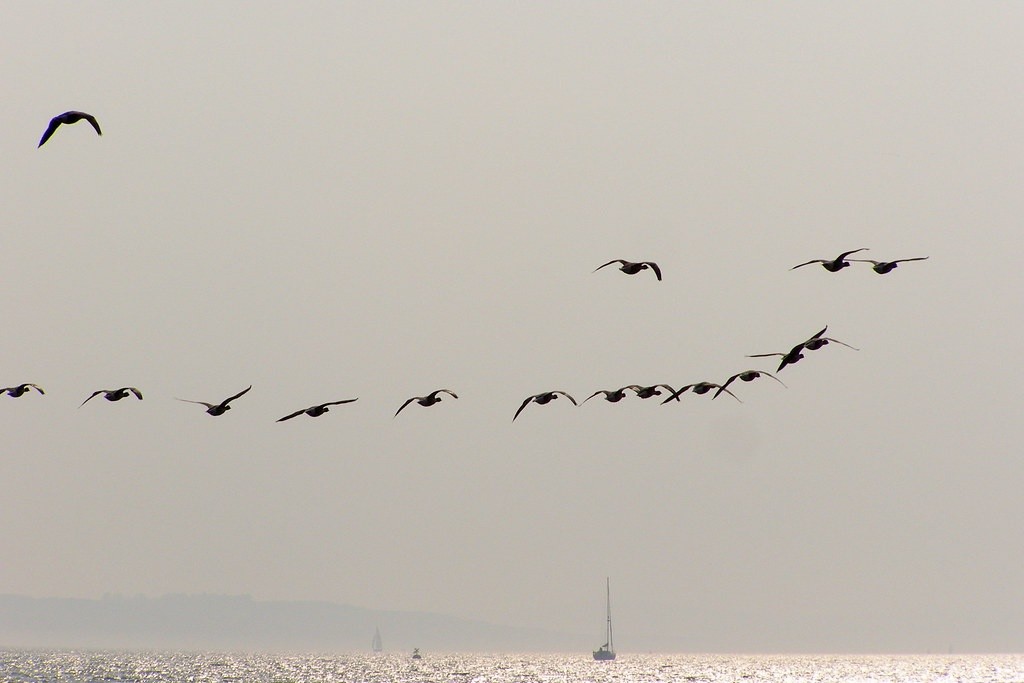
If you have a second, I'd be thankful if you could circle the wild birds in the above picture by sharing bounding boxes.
[591,259,662,281]
[711,370,788,400]
[628,383,681,403]
[37,111,101,149]
[659,381,743,405]
[512,390,578,423]
[0,383,45,398]
[843,255,930,275]
[775,338,859,374]
[78,386,143,409]
[744,325,828,373]
[579,384,642,406]
[174,383,252,416]
[788,248,870,272]
[395,388,459,416]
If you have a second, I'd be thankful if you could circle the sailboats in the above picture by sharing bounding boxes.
[372,626,383,652]
[592,576,617,660]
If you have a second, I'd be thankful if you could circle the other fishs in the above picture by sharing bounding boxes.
[276,397,360,423]
